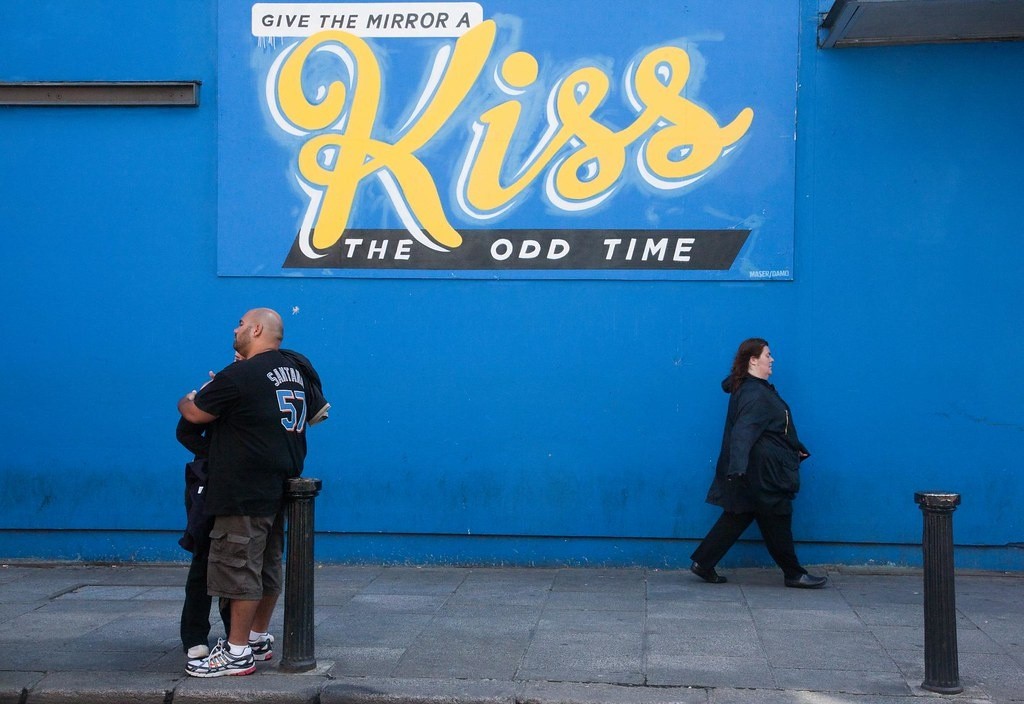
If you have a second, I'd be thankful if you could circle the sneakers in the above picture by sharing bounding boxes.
[184,637,256,677]
[187,644,210,658]
[269,634,275,643]
[217,636,272,660]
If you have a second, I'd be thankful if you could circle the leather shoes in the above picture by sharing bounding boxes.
[785,572,827,589]
[690,561,726,583]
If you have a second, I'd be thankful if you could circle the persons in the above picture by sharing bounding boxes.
[690,338,827,589]
[176,308,307,677]
